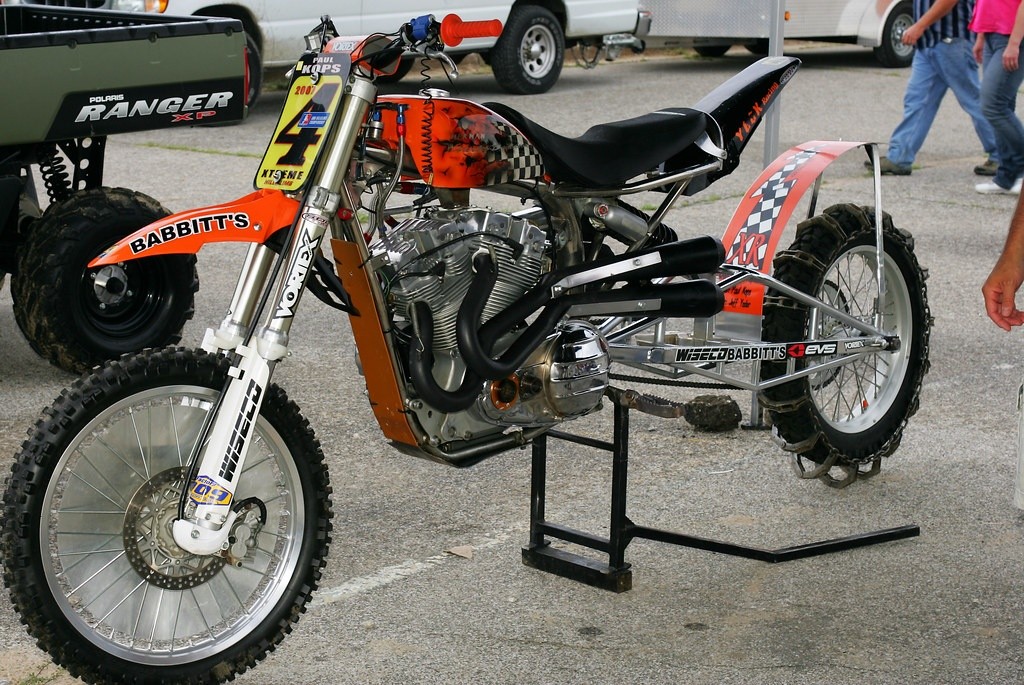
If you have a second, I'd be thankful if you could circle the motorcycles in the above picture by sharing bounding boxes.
[1,15,940,685]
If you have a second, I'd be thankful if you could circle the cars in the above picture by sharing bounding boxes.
[114,1,657,128]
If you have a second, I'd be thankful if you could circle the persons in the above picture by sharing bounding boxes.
[863,0,999,176]
[980,178,1024,332]
[969,0,1024,194]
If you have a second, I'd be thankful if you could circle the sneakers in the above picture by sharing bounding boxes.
[864,156,912,175]
[974,159,1000,175]
[975,177,1023,195]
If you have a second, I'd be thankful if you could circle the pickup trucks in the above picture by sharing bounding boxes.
[1,0,253,378]
